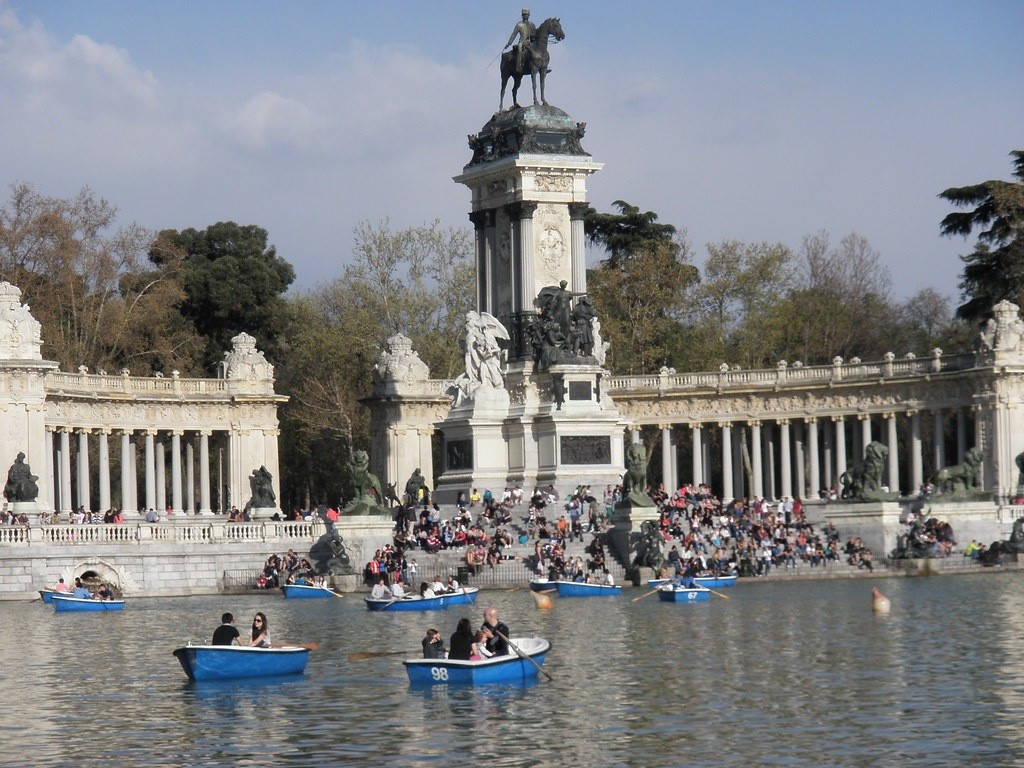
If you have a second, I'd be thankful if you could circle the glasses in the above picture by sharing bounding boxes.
[254,618,261,622]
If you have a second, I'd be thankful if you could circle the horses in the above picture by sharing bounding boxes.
[499,17,565,115]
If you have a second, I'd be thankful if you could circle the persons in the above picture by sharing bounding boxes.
[247,612,272,649]
[901,511,953,556]
[0,506,123,540]
[919,480,934,500]
[453,308,504,407]
[422,608,509,663]
[247,465,276,507]
[880,483,889,494]
[965,540,987,557]
[506,8,551,73]
[141,505,173,534]
[1007,494,1024,506]
[212,612,243,646]
[534,279,598,367]
[406,468,427,504]
[362,483,874,601]
[840,467,862,498]
[292,505,340,523]
[385,482,402,508]
[1010,516,1024,543]
[543,228,560,249]
[5,452,39,501]
[55,576,114,602]
[226,504,251,536]
[257,547,328,591]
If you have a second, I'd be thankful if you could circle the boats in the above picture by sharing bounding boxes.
[172,646,311,680]
[280,585,334,598]
[528,579,557,592]
[50,596,125,611]
[402,635,552,685]
[554,581,623,597]
[656,587,711,603]
[402,587,480,605]
[38,589,94,604]
[647,575,737,589]
[364,594,449,611]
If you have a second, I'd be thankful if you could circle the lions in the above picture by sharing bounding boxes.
[340,449,384,516]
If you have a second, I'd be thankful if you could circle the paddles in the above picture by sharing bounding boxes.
[632,582,675,601]
[459,581,474,604]
[495,629,553,681]
[316,582,344,597]
[506,578,533,592]
[368,649,423,658]
[377,591,412,612]
[538,588,557,594]
[93,585,109,611]
[28,597,41,603]
[263,642,319,651]
[597,584,622,586]
[701,585,728,599]
